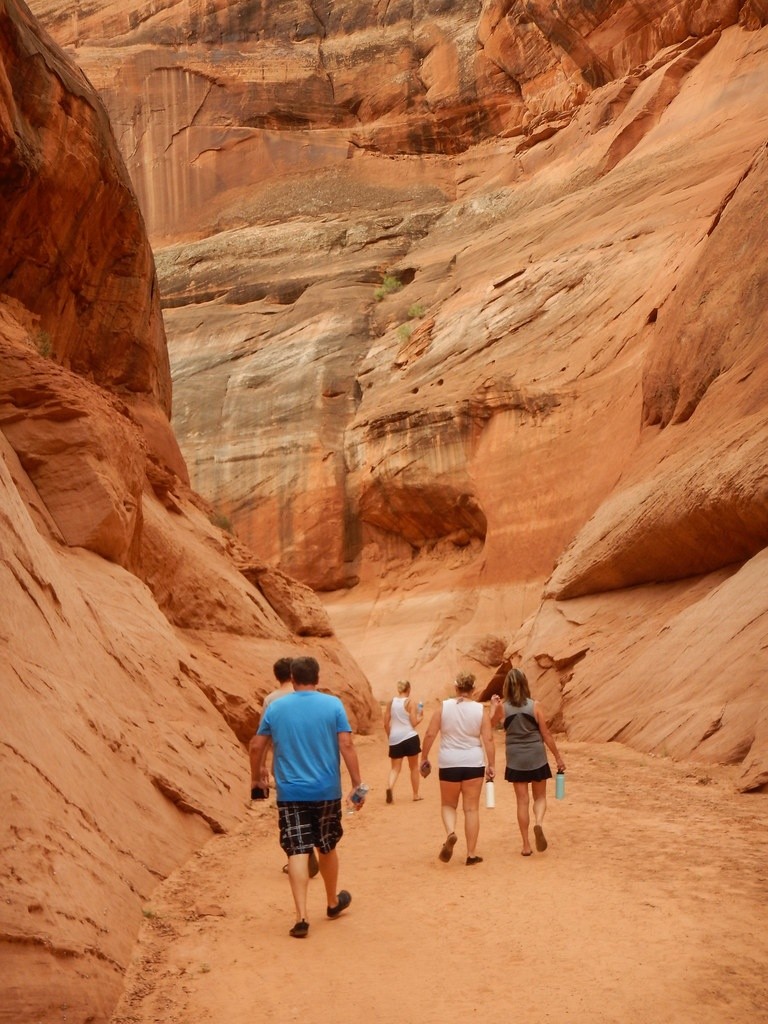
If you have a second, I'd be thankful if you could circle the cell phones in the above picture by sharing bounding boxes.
[422,763,431,778]
[251,787,269,799]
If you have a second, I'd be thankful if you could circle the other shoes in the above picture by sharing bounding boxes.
[327,890,351,917]
[413,796,424,800]
[289,918,309,936]
[386,789,392,803]
[440,832,458,862]
[466,856,483,865]
[308,852,319,878]
[283,864,288,873]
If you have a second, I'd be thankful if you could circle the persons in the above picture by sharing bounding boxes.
[419,671,496,866]
[490,668,567,856]
[384,680,424,804]
[249,656,365,937]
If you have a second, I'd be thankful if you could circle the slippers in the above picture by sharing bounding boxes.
[521,849,534,856]
[534,825,547,853]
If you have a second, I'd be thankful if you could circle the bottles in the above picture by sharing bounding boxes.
[556,769,564,800]
[346,784,368,814]
[486,778,495,808]
[417,701,423,718]
